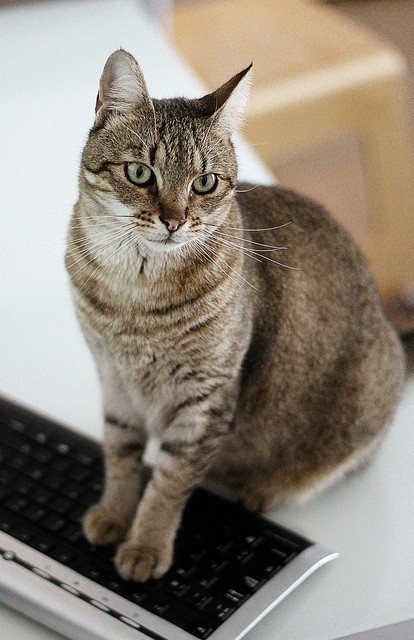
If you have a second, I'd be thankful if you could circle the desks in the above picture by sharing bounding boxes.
[0,0,413,638]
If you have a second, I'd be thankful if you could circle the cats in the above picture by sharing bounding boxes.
[62,45,411,589]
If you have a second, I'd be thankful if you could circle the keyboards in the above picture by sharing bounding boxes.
[0,389,341,640]
